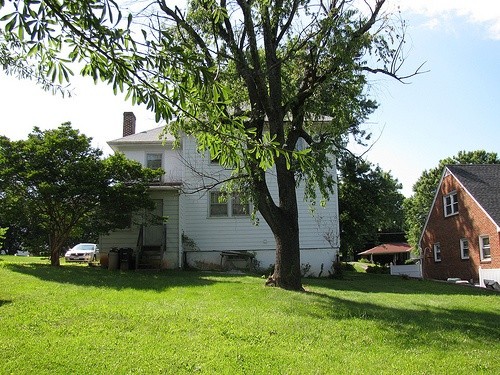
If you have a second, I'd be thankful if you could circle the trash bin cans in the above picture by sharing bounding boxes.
[107,247,119,271]
[118,247,134,272]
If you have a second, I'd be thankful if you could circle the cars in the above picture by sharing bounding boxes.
[65,243,100,263]
